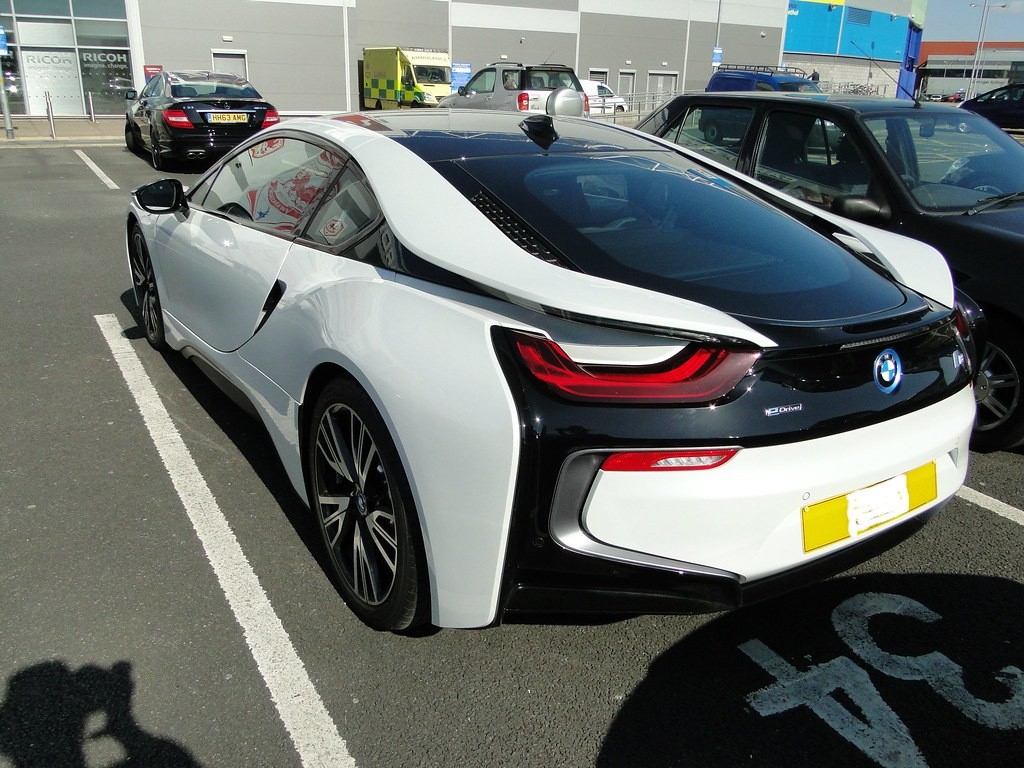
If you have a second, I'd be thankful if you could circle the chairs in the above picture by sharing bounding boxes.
[548,78,559,87]
[530,77,544,88]
[172,87,197,98]
[829,132,874,198]
[215,81,234,94]
[760,124,819,182]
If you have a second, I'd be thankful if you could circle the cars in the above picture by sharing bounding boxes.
[125,70,281,172]
[922,92,965,102]
[125,109,978,638]
[959,83,1024,128]
[102,78,134,100]
[4,72,23,100]
[633,91,1024,453]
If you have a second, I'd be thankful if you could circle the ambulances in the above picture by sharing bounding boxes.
[362,47,451,110]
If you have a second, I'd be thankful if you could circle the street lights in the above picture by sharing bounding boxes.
[963,1,1008,102]
[941,59,970,97]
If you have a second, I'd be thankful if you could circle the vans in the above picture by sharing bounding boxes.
[578,79,627,116]
[698,64,842,154]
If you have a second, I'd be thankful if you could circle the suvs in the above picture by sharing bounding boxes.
[436,62,590,122]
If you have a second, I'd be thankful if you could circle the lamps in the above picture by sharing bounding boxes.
[828,2,837,9]
[890,13,898,19]
